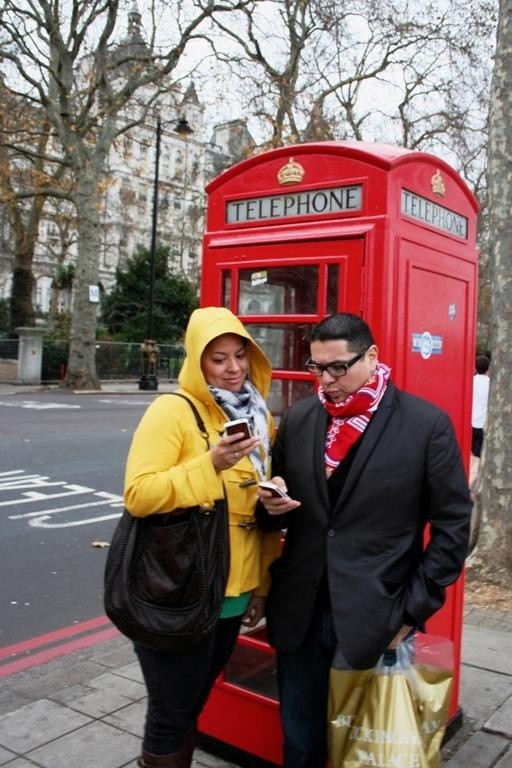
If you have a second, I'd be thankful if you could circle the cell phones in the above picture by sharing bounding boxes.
[224,419,252,447]
[259,482,293,502]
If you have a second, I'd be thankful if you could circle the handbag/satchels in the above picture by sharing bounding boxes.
[102,498,231,645]
[323,634,455,768]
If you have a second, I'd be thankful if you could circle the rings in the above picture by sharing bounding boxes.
[233,452,242,459]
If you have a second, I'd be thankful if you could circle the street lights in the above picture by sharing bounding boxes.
[147,114,194,340]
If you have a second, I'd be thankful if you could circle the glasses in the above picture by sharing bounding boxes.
[303,354,364,379]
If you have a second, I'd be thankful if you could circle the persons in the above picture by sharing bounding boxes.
[124,303,283,768]
[469,352,490,490]
[255,311,475,768]
